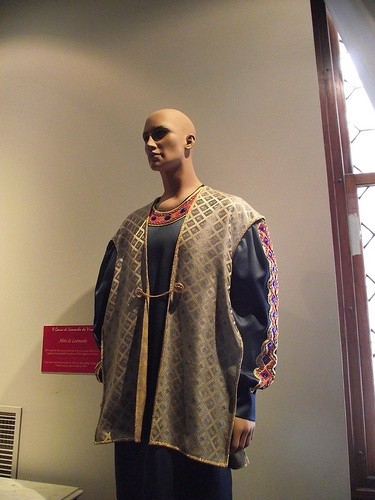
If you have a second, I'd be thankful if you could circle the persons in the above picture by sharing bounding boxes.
[90,109,280,500]
